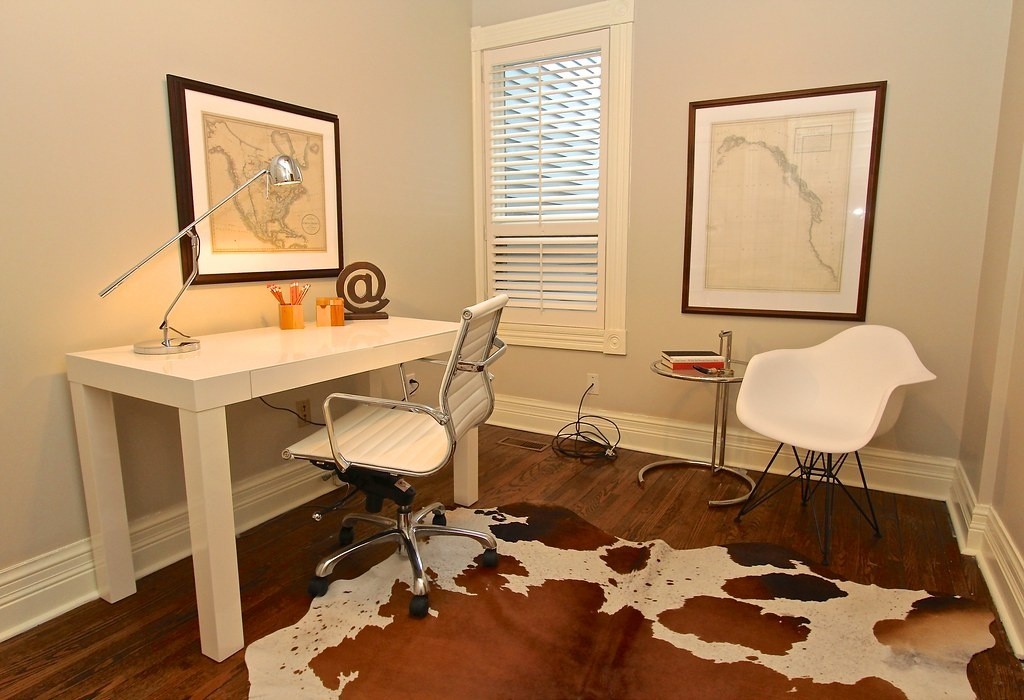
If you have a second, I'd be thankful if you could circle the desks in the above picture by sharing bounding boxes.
[637,328,757,507]
[66,315,480,663]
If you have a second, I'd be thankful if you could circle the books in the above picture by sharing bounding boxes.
[660,350,726,370]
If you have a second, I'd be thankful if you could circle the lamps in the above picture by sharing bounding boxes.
[100,153,303,356]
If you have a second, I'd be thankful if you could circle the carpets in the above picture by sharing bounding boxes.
[240,502,998,700]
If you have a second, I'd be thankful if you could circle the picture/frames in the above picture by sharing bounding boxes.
[680,78,889,324]
[165,72,344,285]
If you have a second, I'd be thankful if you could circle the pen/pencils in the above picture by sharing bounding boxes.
[289,281,312,306]
[267,282,285,306]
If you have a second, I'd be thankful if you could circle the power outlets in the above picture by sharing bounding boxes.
[405,373,417,395]
[587,373,599,395]
[295,398,312,427]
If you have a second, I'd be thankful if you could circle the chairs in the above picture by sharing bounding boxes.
[735,323,939,569]
[277,293,511,617]
